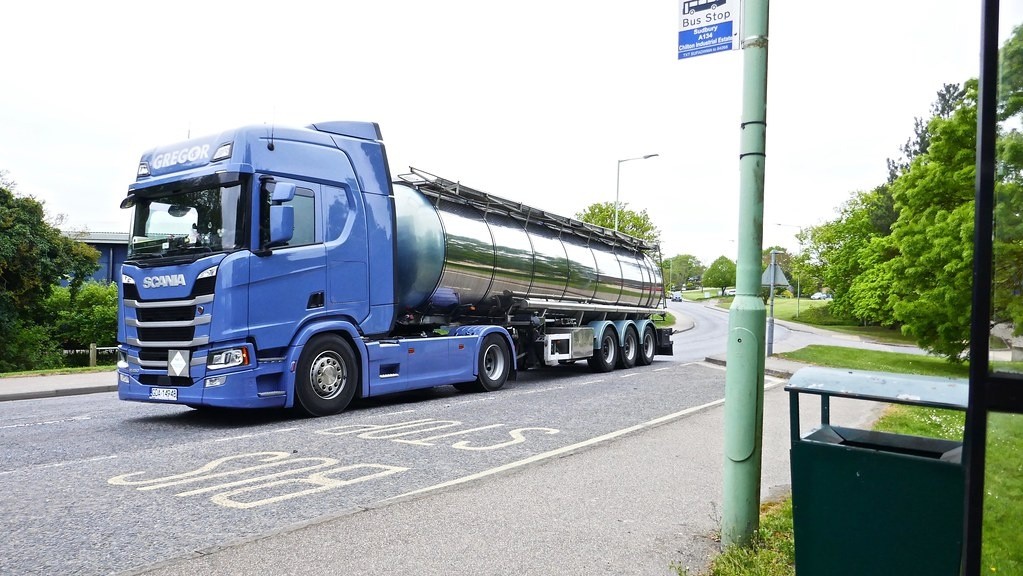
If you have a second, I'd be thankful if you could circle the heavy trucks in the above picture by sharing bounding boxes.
[115,119,675,417]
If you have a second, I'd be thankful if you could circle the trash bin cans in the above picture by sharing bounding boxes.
[783,365,970,576]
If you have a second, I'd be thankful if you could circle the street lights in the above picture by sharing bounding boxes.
[777,223,800,319]
[767,249,784,356]
[613,153,660,230]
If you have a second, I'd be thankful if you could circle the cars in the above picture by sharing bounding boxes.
[671,292,682,302]
[666,291,672,299]
[726,289,735,296]
[811,293,827,300]
